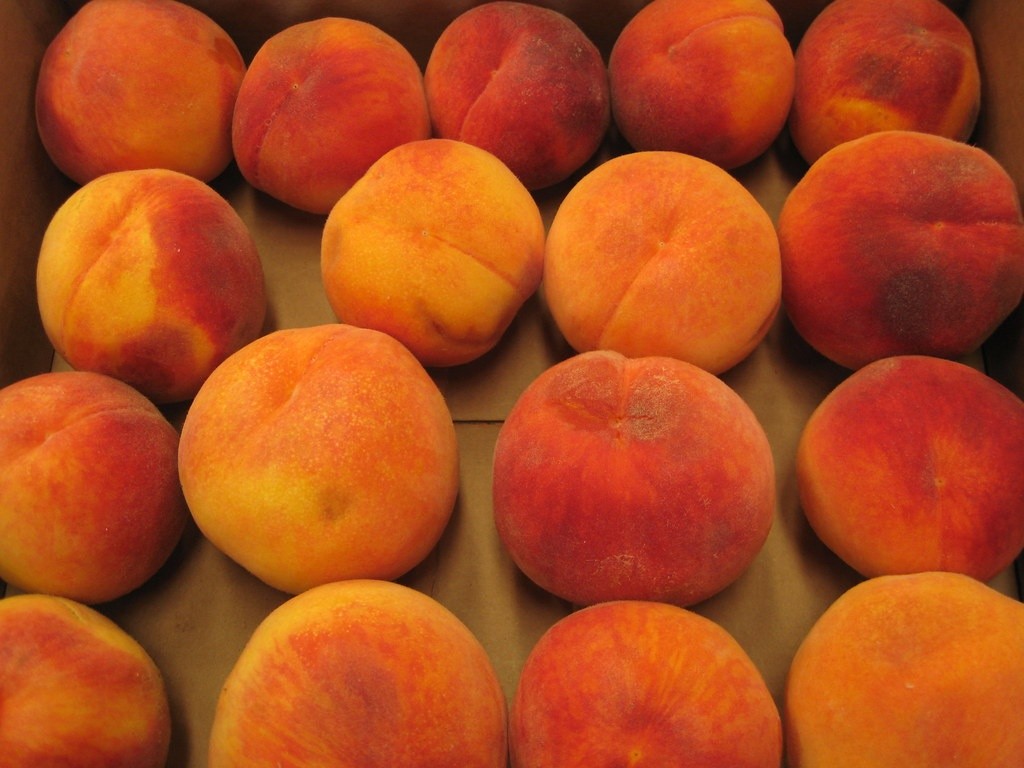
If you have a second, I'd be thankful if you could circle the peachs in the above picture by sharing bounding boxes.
[0,0,1024,768]
